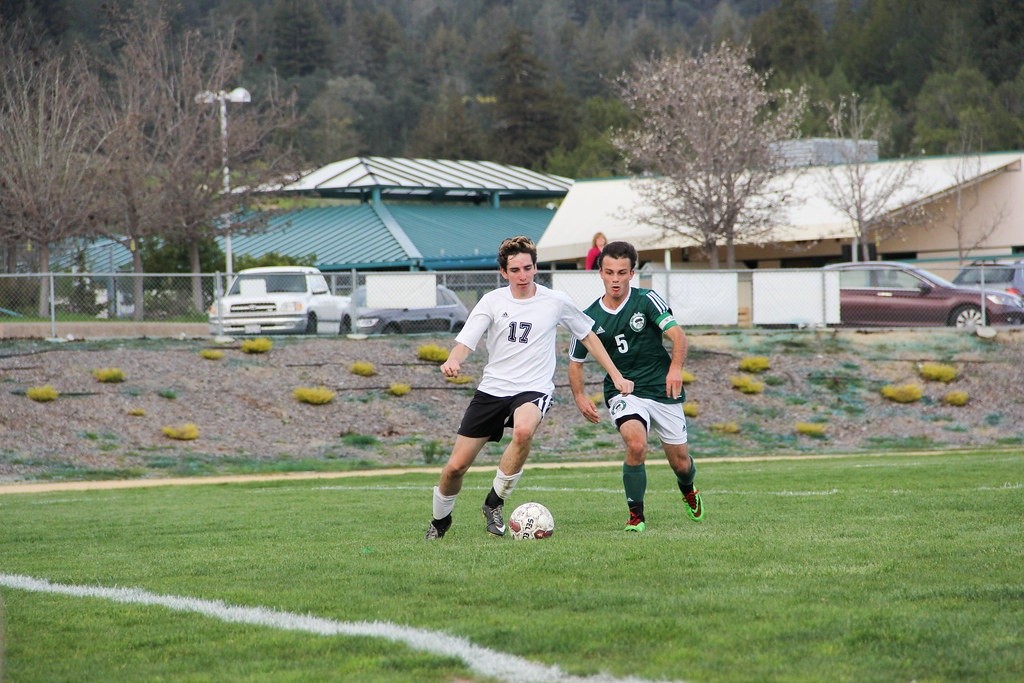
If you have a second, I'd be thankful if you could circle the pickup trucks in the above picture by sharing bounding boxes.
[209,263,351,338]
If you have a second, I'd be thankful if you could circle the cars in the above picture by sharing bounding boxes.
[338,280,470,336]
[952,257,1023,303]
[791,260,1024,331]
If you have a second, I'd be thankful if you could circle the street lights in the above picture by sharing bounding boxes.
[195,85,252,287]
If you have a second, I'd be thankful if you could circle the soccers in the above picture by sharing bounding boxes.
[508,502,555,541]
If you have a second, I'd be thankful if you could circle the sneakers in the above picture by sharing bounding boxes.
[682,485,704,522]
[482,502,506,536]
[624,514,645,533]
[424,516,453,541]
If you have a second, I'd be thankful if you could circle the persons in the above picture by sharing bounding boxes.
[424,234,635,542]
[585,232,607,270]
[568,241,703,534]
